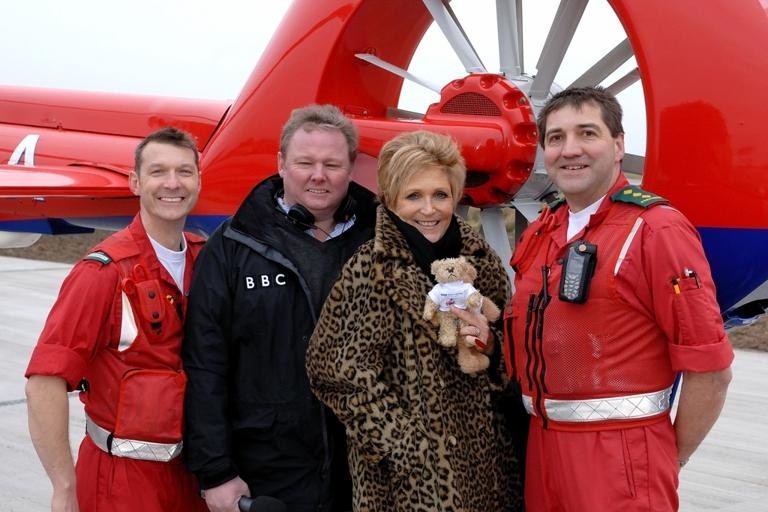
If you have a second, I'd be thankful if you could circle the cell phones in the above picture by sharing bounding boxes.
[563,251,586,301]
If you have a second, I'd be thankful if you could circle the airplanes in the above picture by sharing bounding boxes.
[0,0,768,335]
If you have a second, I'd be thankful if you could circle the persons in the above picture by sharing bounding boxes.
[177,96,389,512]
[23,129,210,512]
[490,86,737,510]
[302,130,522,512]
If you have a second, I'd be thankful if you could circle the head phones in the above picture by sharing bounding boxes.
[274,188,359,231]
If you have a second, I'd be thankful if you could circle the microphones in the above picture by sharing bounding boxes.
[198,489,287,512]
